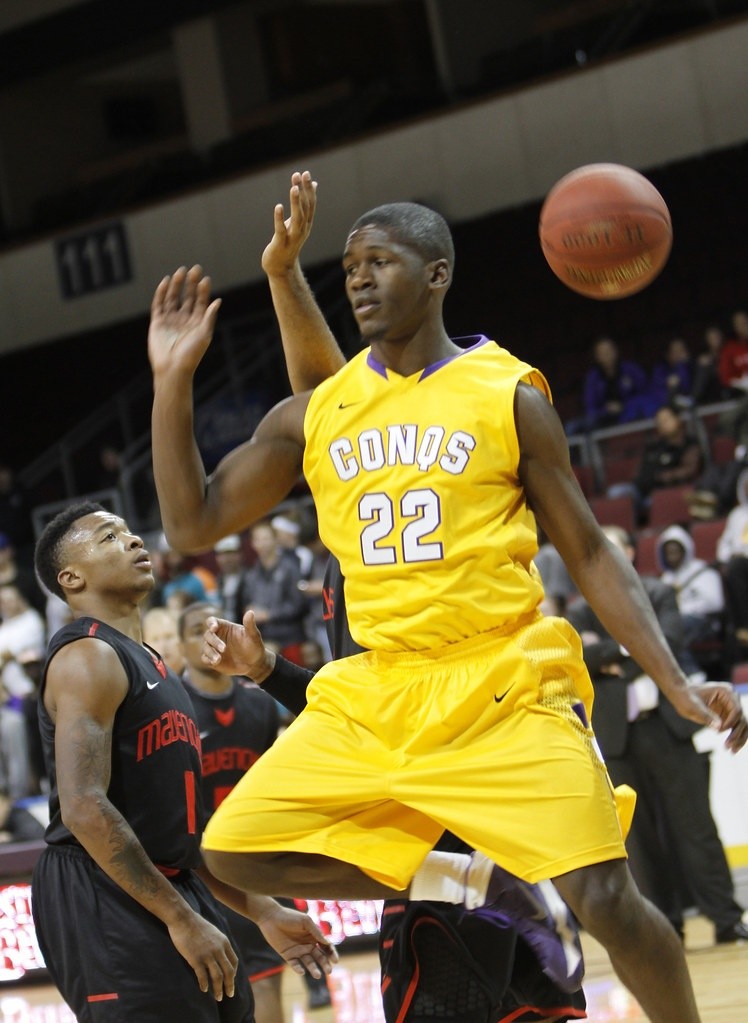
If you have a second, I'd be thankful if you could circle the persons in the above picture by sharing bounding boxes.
[201,170,588,1022]
[147,200,747,1023]
[0,311,747,1023]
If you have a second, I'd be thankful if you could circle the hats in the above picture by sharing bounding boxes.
[213,533,241,553]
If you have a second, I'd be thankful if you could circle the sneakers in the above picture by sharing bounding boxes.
[462,847,585,993]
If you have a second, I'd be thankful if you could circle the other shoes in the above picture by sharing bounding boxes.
[302,965,329,1009]
[716,919,748,944]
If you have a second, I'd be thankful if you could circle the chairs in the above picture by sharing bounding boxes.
[580,425,748,680]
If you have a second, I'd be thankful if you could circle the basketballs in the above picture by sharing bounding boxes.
[540,162,674,301]
